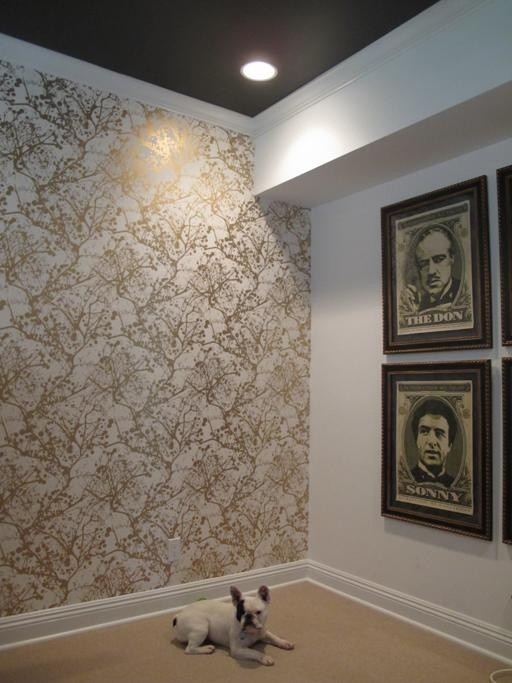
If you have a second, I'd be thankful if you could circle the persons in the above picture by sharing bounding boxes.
[398,226,462,313]
[403,401,465,489]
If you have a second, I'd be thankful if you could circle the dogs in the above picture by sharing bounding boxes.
[169,584,295,667]
